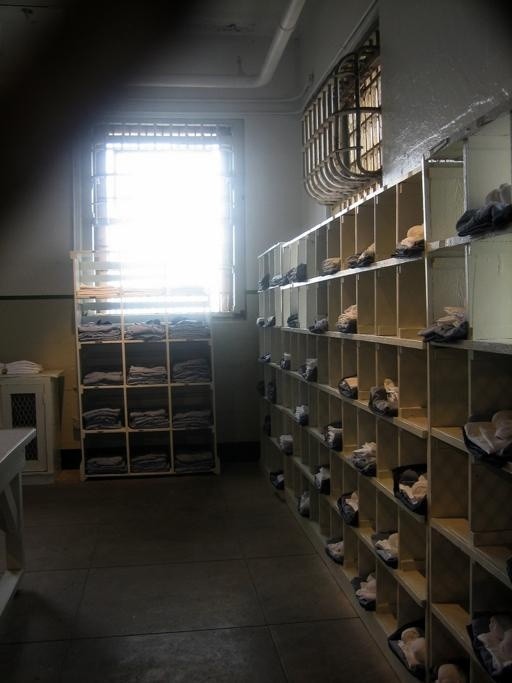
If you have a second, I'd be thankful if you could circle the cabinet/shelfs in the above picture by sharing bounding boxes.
[256,143,512,682]
[0,376,59,485]
[72,250,222,481]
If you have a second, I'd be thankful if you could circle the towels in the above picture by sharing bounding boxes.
[1,360,44,375]
[257,181,512,681]
[76,285,213,476]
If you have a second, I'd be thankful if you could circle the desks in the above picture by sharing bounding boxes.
[0,428,38,619]
